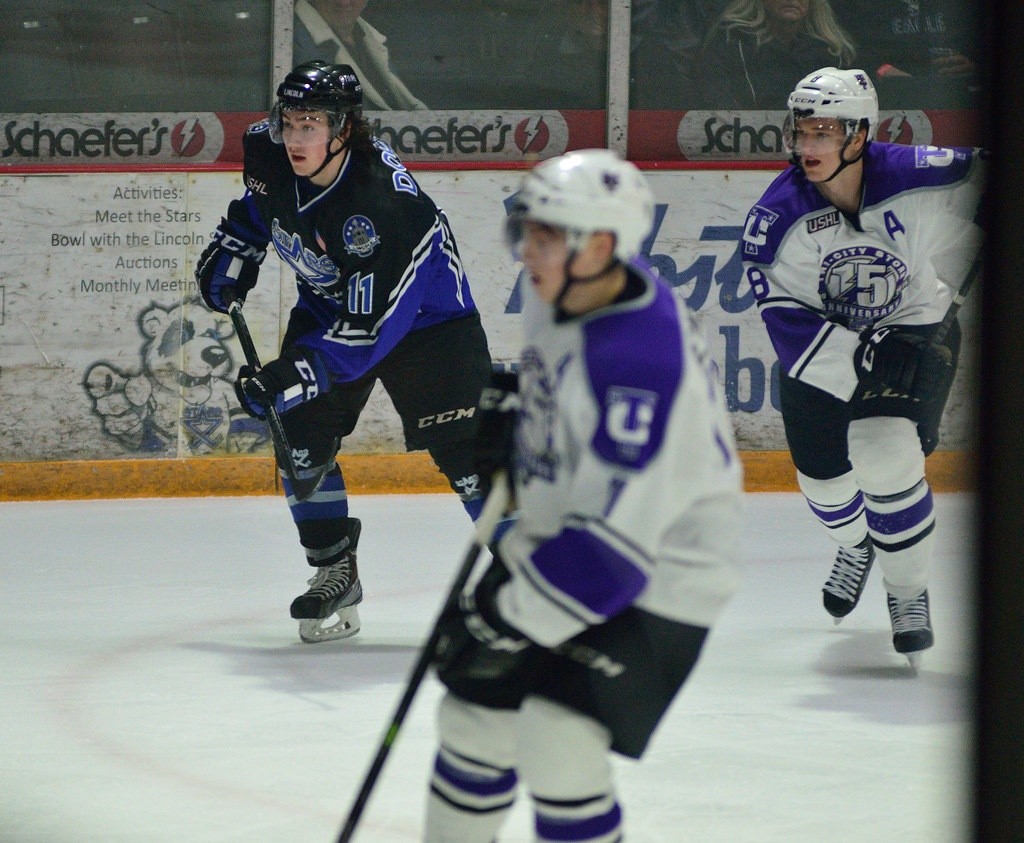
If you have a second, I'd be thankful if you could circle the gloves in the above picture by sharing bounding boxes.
[233,344,322,421]
[472,371,521,516]
[431,580,537,703]
[853,327,953,401]
[194,216,268,314]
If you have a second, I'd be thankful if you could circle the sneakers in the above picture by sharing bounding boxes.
[822,532,877,626]
[886,588,935,669]
[289,517,363,646]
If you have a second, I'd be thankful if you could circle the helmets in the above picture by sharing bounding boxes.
[276,60,364,135]
[509,147,656,265]
[787,66,879,143]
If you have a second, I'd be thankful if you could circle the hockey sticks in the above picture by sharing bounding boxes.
[931,241,987,346]
[333,468,511,842]
[225,291,343,505]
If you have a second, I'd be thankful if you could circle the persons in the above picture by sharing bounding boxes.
[292,1,981,109]
[741,68,986,671]
[417,144,744,842]
[197,61,514,644]
